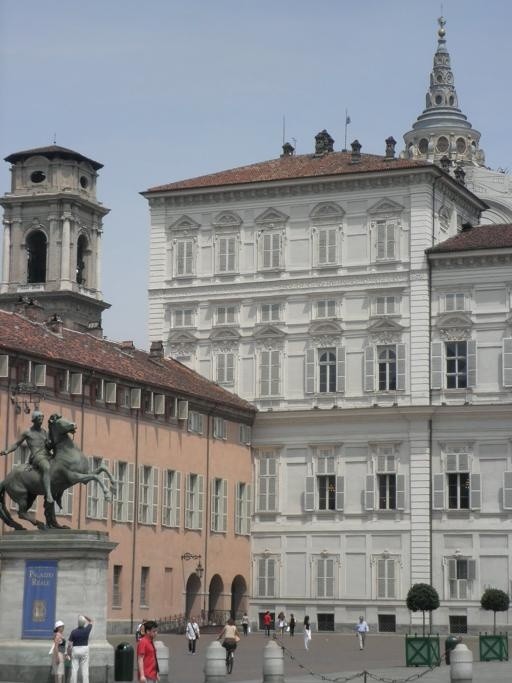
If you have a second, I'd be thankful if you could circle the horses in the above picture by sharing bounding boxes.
[0,414,115,531]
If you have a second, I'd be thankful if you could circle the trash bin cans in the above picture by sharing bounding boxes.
[115,641,134,682]
[445,636,460,665]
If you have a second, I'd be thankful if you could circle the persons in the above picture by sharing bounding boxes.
[241,612,248,637]
[136,619,147,642]
[303,616,311,651]
[278,612,287,638]
[50,621,70,683]
[0,411,63,510]
[186,616,200,656]
[217,618,240,651]
[263,611,273,638]
[355,616,369,650]
[288,614,295,637]
[137,621,160,683]
[67,615,93,683]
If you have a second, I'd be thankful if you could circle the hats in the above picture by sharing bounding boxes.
[55,621,64,628]
[78,615,86,626]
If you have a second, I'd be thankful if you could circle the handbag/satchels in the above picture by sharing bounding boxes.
[235,635,240,642]
[48,643,55,655]
[195,633,199,639]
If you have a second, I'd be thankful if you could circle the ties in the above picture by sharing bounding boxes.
[151,641,159,672]
